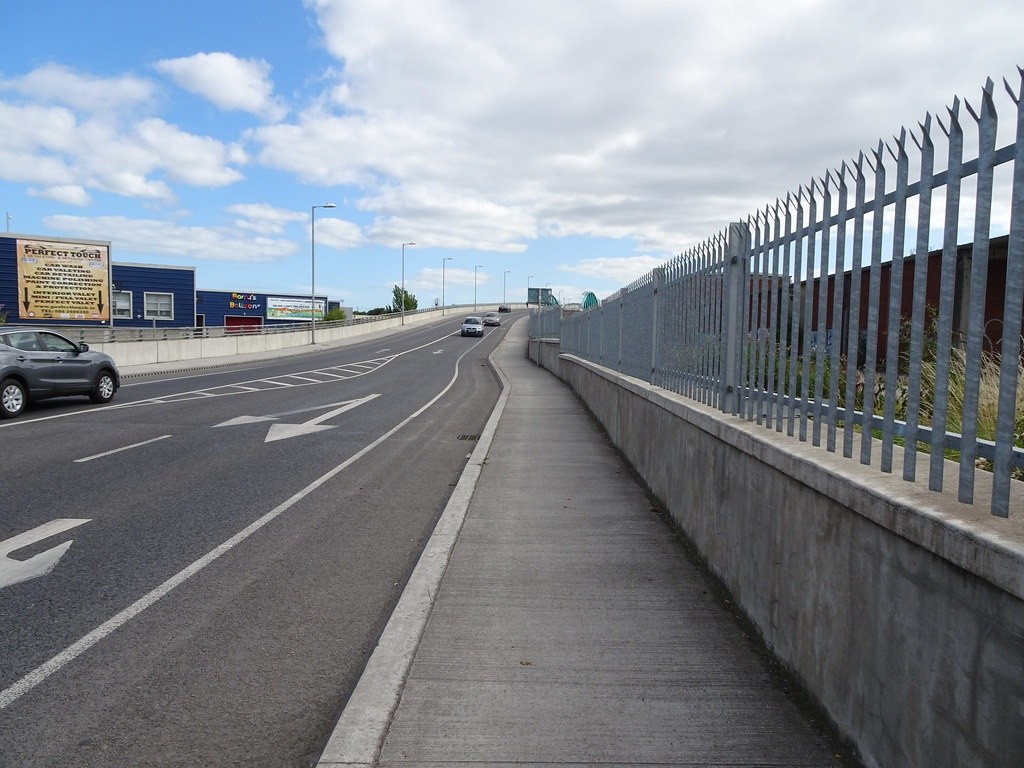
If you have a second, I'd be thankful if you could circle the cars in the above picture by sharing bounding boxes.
[482,312,501,326]
[499,304,511,312]
[461,317,484,337]
[0,331,121,417]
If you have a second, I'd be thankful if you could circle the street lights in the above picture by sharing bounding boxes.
[402,243,416,326]
[546,283,550,288]
[311,203,336,345]
[504,270,511,304]
[475,266,482,311]
[443,258,453,316]
[528,275,533,289]
